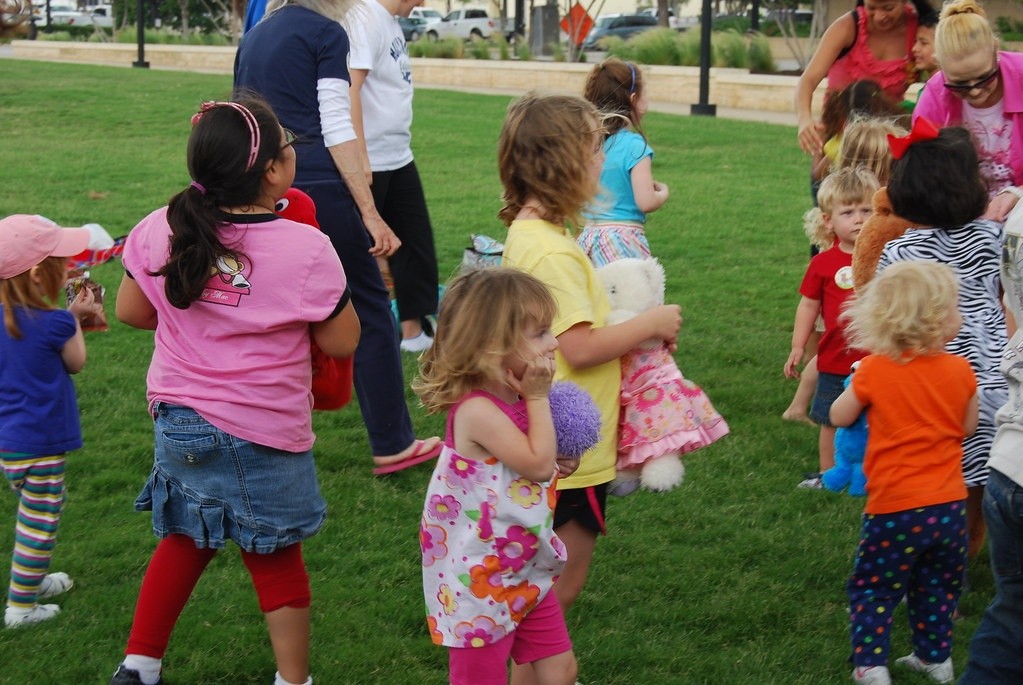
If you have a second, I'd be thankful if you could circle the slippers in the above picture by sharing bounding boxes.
[373,440,445,476]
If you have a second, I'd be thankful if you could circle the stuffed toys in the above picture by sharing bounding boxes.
[596,257,729,497]
[275,188,355,407]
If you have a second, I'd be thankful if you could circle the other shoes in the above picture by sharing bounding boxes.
[796,472,824,489]
[110,664,170,685]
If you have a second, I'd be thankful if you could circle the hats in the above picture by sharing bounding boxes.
[0,214,93,276]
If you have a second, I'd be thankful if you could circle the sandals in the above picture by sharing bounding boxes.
[4,603,61,629]
[37,571,74,600]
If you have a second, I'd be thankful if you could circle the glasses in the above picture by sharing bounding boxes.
[940,48,1000,93]
[276,127,298,152]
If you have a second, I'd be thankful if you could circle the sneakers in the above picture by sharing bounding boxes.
[852,665,893,685]
[895,651,955,684]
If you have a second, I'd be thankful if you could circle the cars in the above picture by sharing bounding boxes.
[582,14,658,54]
[394,6,445,41]
[425,8,494,42]
[29,0,117,37]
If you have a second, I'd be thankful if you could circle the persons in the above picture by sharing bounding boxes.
[231,1,1023,685]
[0,214,95,628]
[111,90,362,685]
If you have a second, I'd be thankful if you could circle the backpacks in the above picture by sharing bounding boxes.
[459,232,504,275]
